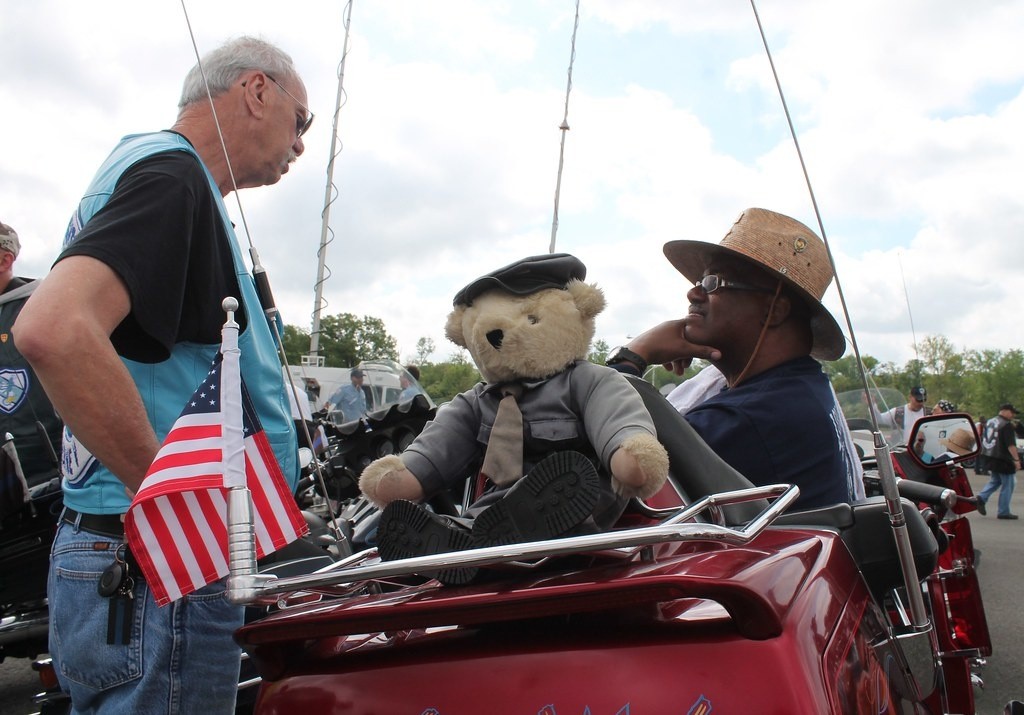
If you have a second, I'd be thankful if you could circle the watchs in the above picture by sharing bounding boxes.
[605,346,647,373]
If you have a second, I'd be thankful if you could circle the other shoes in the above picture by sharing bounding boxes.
[974,495,986,515]
[997,513,1018,519]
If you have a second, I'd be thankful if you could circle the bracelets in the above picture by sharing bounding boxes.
[1014,459,1020,461]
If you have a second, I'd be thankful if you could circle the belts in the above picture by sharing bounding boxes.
[63,509,127,539]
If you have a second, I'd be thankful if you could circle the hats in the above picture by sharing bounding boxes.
[663,207,845,360]
[911,386,927,400]
[938,429,975,456]
[1000,403,1020,414]
[0,222,20,261]
[351,370,365,377]
[938,399,954,414]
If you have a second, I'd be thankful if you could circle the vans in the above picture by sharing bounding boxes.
[285,365,399,417]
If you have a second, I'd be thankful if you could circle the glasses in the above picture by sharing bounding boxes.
[918,438,924,442]
[695,277,776,293]
[915,398,925,403]
[242,74,314,137]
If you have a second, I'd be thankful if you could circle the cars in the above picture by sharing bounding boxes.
[959,416,1024,470]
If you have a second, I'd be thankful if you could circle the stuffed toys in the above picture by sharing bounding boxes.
[357,253,669,584]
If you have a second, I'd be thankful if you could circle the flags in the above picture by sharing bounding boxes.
[122,352,310,607]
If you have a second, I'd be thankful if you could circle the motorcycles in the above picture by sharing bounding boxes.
[0,279,993,715]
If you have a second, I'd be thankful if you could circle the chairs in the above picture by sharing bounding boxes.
[463,373,939,626]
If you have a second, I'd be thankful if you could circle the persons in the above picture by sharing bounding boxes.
[975,416,990,475]
[15,36,316,715]
[864,387,933,443]
[324,369,367,422]
[976,404,1021,519]
[914,428,975,464]
[603,209,863,512]
[1012,419,1024,470]
[0,223,58,489]
[931,400,954,414]
[397,365,420,401]
